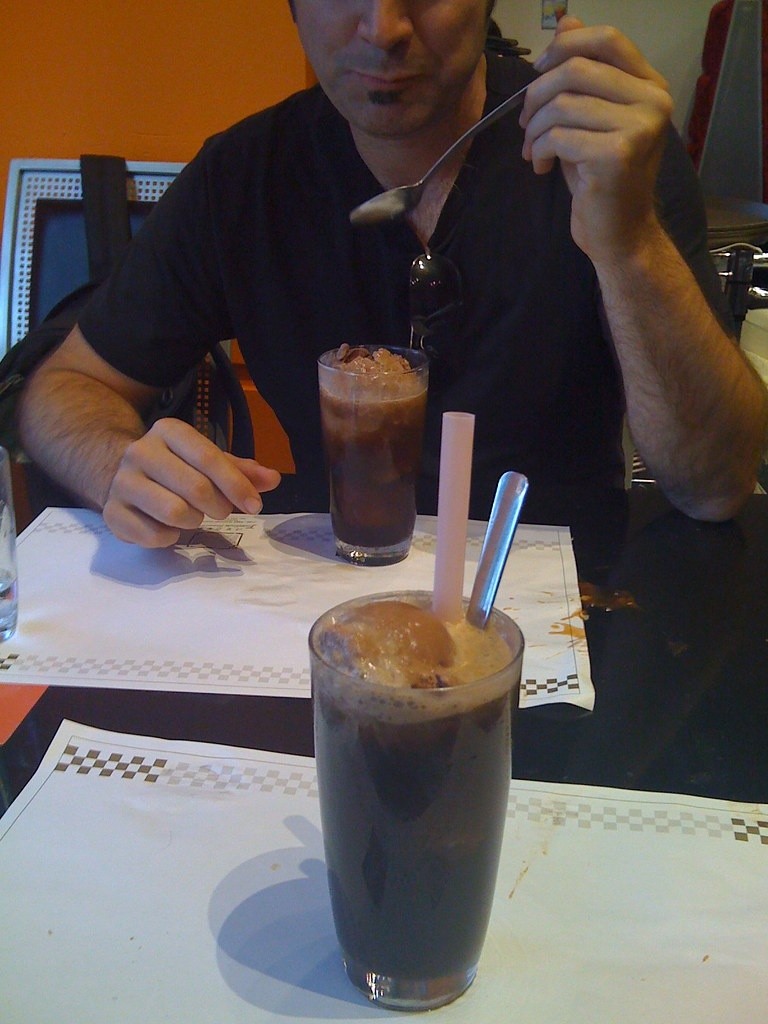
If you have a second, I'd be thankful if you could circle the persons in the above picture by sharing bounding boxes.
[14,0,768,548]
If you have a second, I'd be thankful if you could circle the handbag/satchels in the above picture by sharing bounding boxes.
[0,153,255,463]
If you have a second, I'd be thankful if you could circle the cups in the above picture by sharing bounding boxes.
[308,590,525,1011]
[0,446,17,640]
[317,345,430,566]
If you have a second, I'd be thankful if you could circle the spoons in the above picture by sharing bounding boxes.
[350,86,528,236]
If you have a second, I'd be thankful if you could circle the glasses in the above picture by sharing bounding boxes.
[408,245,466,397]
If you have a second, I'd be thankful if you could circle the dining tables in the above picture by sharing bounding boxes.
[0,489,768,1024]
